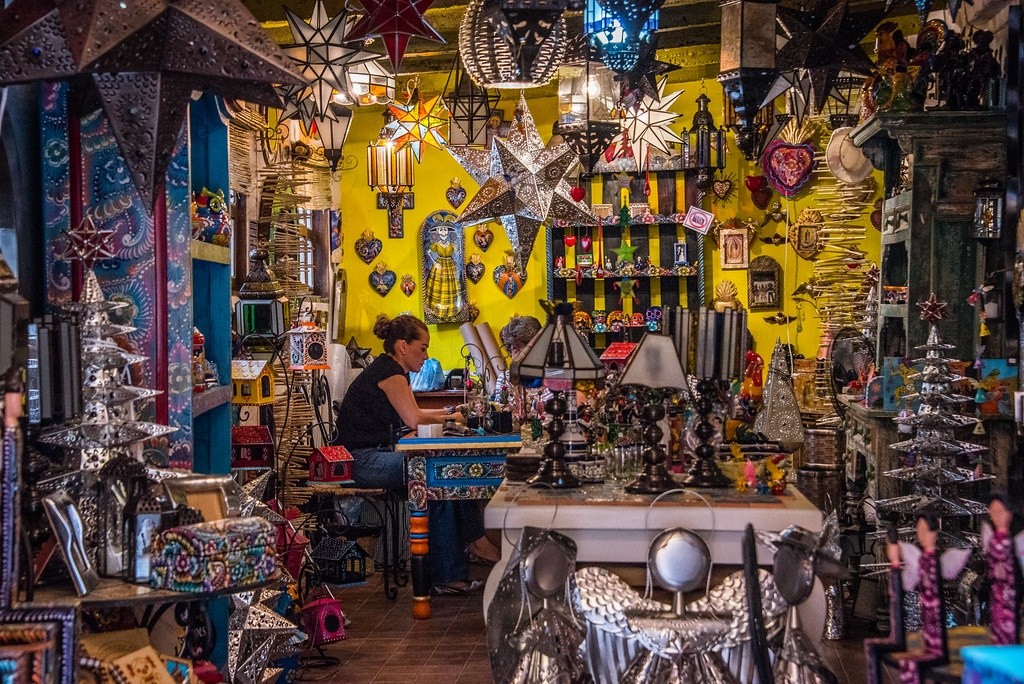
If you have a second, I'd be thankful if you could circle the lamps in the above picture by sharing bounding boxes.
[614,333,696,495]
[272,0,776,221]
[509,314,604,491]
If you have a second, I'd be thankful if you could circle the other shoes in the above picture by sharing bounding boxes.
[435,575,486,595]
[464,548,501,565]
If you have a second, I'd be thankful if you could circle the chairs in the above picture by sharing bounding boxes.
[311,374,408,600]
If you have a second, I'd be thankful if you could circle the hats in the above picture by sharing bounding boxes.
[825,126,875,184]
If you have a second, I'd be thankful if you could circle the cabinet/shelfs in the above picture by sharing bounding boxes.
[231,295,338,608]
[836,109,1018,526]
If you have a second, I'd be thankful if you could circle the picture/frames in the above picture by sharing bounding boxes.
[720,229,748,269]
[747,267,779,309]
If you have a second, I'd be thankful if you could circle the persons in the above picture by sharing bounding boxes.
[341,314,474,595]
[494,314,585,414]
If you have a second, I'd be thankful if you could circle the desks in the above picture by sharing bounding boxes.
[395,430,523,620]
[483,477,827,650]
